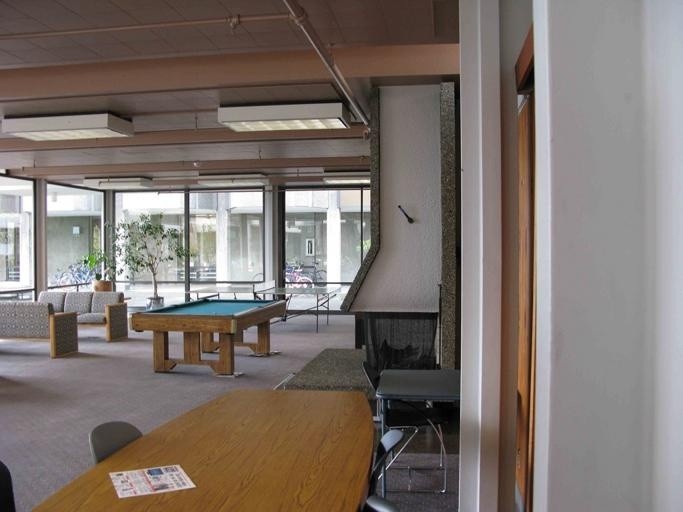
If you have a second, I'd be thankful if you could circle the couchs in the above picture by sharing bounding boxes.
[38,290,128,343]
[0,300,79,358]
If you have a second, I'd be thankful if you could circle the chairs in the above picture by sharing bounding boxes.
[360,360,447,494]
[88,421,142,464]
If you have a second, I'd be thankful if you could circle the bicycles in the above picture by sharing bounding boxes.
[282,255,327,289]
[54,259,95,288]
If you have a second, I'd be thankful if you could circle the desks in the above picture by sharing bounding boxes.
[375,368,460,497]
[29,389,374,511]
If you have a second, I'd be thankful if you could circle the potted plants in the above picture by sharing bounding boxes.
[80,253,112,292]
[106,214,197,309]
